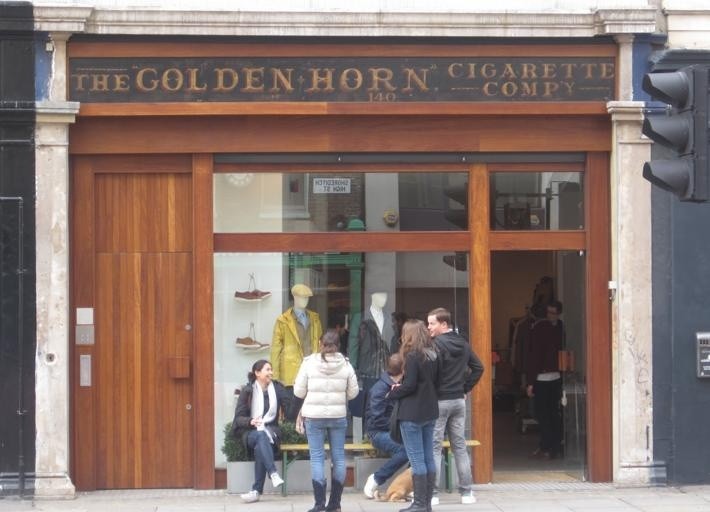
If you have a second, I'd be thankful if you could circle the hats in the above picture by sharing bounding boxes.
[291,284,314,298]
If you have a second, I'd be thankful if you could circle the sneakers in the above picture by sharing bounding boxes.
[363,473,380,497]
[460,493,476,504]
[270,473,284,488]
[239,490,260,503]
[410,497,440,505]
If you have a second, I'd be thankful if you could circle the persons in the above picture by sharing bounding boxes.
[294,326,363,512]
[426,307,483,505]
[544,300,565,347]
[233,358,296,505]
[361,353,409,500]
[346,277,398,444]
[271,282,323,424]
[387,320,440,512]
[523,302,565,462]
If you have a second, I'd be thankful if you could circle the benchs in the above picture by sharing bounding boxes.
[279,439,483,496]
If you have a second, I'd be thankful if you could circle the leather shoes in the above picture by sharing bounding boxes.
[529,448,551,461]
[234,291,263,303]
[234,388,240,396]
[236,337,261,348]
[553,453,564,459]
[252,289,272,298]
[244,342,270,353]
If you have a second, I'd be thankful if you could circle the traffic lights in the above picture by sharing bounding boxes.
[438,176,468,271]
[640,66,709,203]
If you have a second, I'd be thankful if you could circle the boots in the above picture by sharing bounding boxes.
[309,479,329,511]
[399,475,429,511]
[426,471,436,511]
[325,479,345,512]
